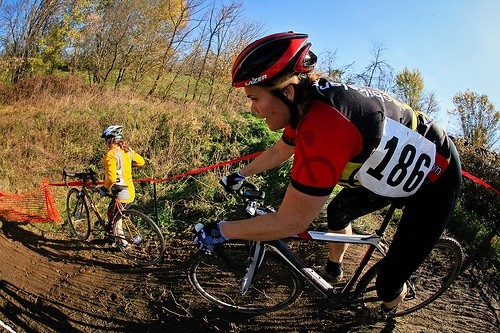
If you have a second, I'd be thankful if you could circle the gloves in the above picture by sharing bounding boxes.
[219,171,246,191]
[191,220,228,253]
[100,186,108,198]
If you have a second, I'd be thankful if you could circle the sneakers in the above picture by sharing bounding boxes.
[365,303,397,322]
[309,265,343,282]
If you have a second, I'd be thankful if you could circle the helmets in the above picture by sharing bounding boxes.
[101,125,123,138]
[231,30,318,88]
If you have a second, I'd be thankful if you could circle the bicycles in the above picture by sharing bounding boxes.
[185,181,464,316]
[63,165,165,266]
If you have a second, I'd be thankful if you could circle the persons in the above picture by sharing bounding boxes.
[99,126,146,247]
[188,32,462,325]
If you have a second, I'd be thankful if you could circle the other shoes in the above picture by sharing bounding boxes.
[113,239,128,247]
